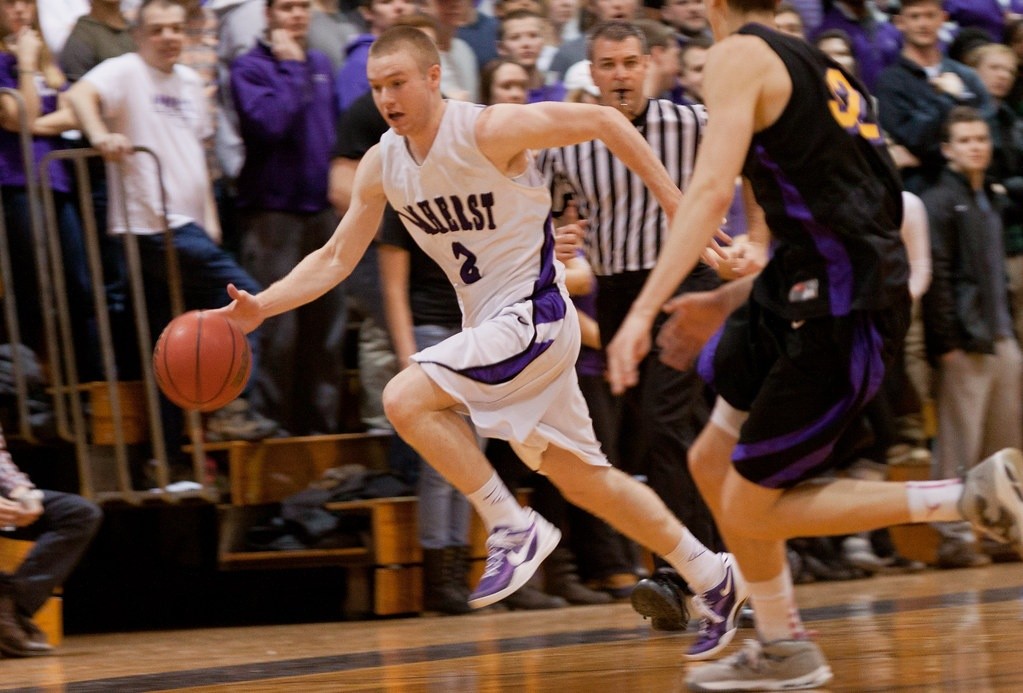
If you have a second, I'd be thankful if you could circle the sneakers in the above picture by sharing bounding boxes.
[957,446,1023,558]
[683,630,833,693]
[680,551,750,661]
[630,566,757,633]
[467,505,563,612]
[208,397,279,441]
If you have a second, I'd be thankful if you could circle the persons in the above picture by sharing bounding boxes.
[0,0,1022,616]
[204,25,751,663]
[534,20,772,632]
[604,0,1023,693]
[0,428,105,658]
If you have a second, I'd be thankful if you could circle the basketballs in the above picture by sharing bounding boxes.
[153,310,251,413]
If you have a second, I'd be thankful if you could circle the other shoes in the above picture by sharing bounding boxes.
[786,537,993,583]
[495,548,649,610]
[1,596,56,655]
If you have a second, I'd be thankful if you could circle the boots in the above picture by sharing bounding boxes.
[420,541,474,616]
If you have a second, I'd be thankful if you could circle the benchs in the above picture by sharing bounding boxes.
[45,377,538,615]
